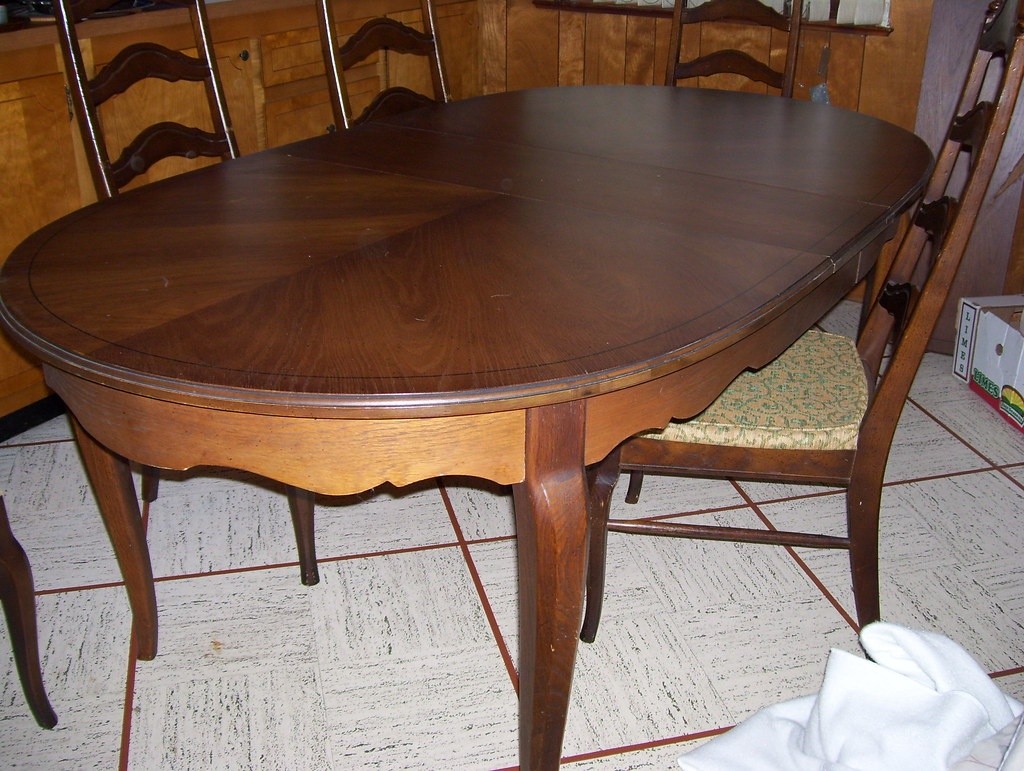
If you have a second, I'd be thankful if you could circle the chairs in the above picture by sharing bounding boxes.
[580,0,1024,663]
[52,0,322,586]
[317,0,449,132]
[665,0,804,97]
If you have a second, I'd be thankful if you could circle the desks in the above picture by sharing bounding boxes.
[0,83,938,771]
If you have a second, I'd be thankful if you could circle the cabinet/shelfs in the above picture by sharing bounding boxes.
[0,0,936,445]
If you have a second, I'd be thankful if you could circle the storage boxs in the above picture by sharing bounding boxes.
[951,294,1024,435]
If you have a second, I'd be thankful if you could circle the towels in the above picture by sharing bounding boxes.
[673,620,1024,771]
